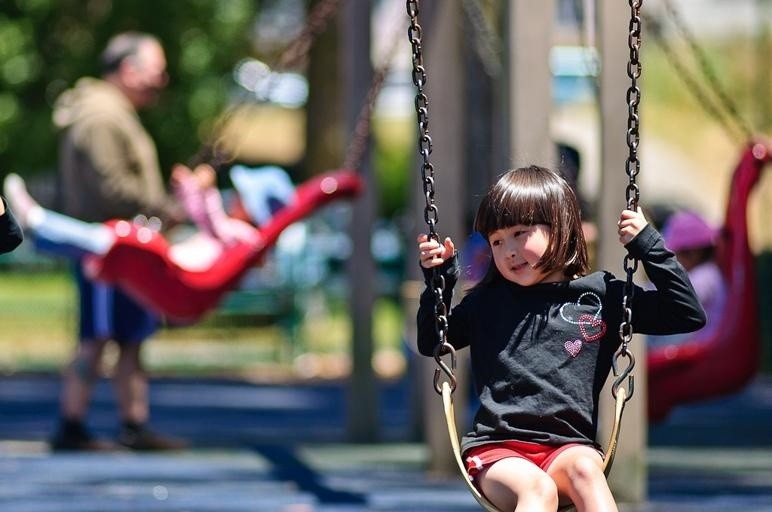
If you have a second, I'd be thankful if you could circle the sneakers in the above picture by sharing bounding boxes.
[47,416,99,454]
[117,416,195,452]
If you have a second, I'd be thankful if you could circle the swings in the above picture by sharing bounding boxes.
[573,2,772,425]
[405,0,643,511]
[81,1,411,327]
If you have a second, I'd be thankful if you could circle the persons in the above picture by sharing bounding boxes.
[0,196,23,255]
[418,166,705,512]
[648,208,723,349]
[49,30,181,451]
[4,163,294,274]
